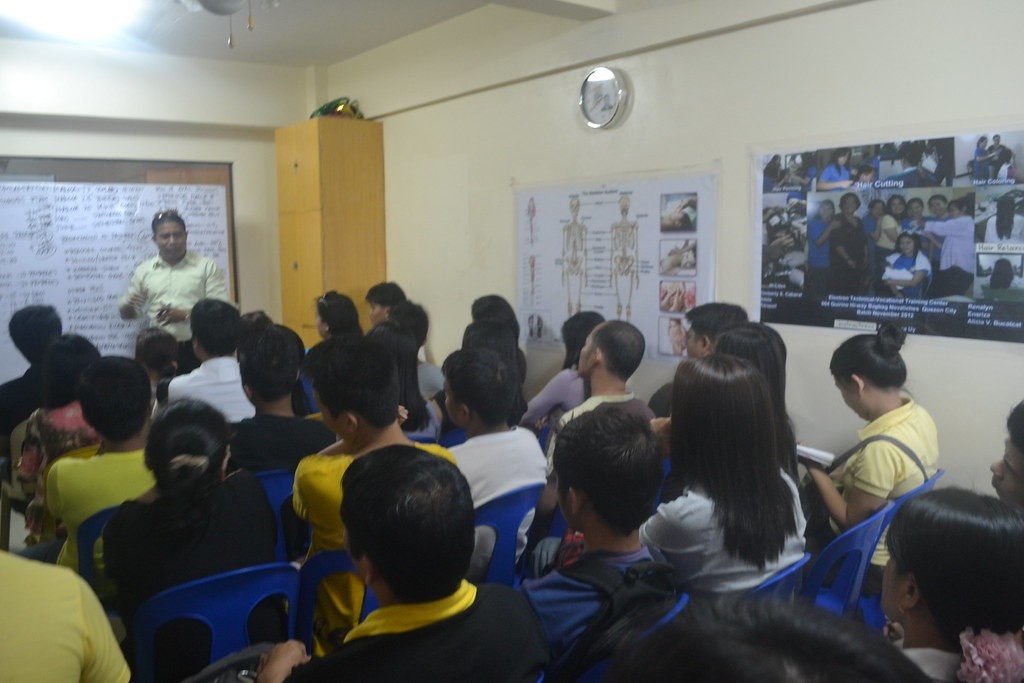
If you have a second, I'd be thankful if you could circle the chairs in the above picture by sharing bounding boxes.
[71,370,941,683]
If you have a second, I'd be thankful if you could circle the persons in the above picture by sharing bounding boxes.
[809,189,975,299]
[879,138,956,188]
[973,135,1024,184]
[984,195,1024,244]
[984,258,1024,288]
[817,148,877,191]
[761,191,807,246]
[668,318,689,356]
[762,154,816,193]
[117,214,231,375]
[0,279,1024,682]
[660,193,697,314]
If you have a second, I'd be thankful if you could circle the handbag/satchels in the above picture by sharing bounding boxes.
[797,474,844,589]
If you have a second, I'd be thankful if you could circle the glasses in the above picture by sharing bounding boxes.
[153,211,178,221]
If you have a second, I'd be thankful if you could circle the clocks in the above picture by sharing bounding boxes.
[578,66,628,130]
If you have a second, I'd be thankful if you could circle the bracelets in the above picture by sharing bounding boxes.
[183,308,190,325]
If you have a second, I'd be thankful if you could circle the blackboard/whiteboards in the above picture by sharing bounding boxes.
[0,181,228,386]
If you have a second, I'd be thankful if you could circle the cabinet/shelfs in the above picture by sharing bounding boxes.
[274,115,386,351]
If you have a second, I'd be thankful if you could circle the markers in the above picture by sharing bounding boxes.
[156,303,170,316]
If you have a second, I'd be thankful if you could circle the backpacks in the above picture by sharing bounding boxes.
[560,558,686,680]
